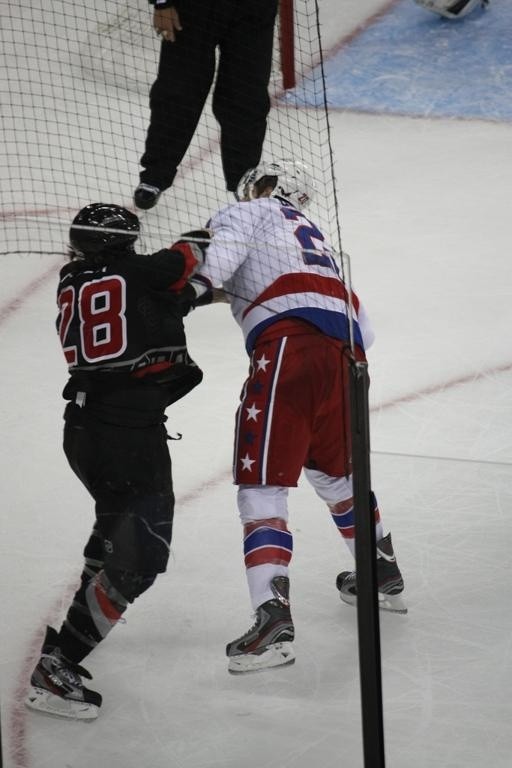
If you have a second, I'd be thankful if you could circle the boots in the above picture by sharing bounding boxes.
[335,532,405,595]
[225,573,294,660]
[31,625,103,708]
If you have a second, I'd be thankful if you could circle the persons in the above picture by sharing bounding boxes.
[183,156,403,656]
[28,204,230,708]
[132,2,293,210]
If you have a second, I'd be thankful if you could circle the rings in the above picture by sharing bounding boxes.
[159,30,168,36]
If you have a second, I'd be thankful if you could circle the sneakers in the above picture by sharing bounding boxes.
[133,180,169,210]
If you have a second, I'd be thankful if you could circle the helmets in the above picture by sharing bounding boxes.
[68,202,141,256]
[237,161,316,213]
